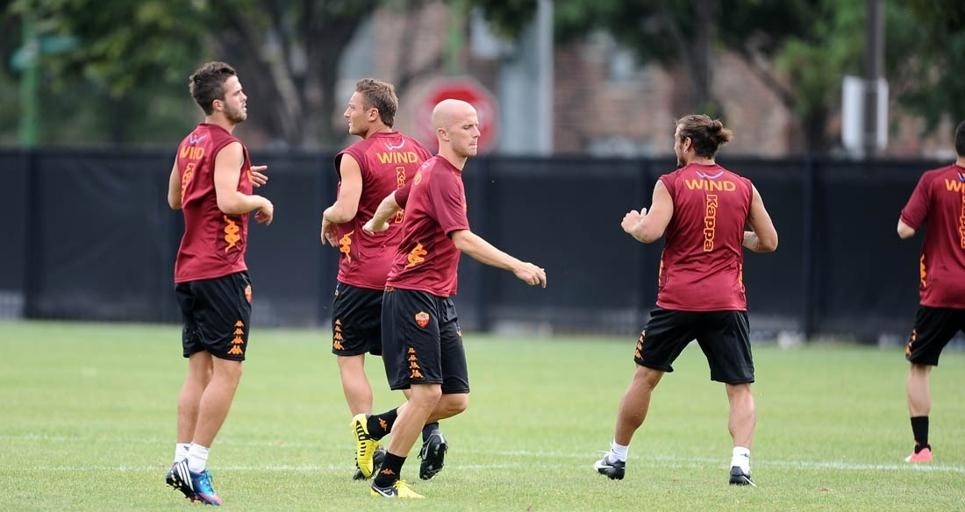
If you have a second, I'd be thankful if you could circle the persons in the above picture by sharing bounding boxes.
[592,110,780,486]
[166,59,275,504]
[897,125,965,464]
[349,98,547,499]
[321,79,448,480]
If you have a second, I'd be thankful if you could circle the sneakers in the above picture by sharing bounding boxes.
[352,413,383,479]
[369,478,424,500]
[166,462,199,502]
[593,455,626,480]
[905,443,934,464]
[352,446,388,480]
[416,429,448,480]
[172,458,223,506]
[729,466,753,486]
